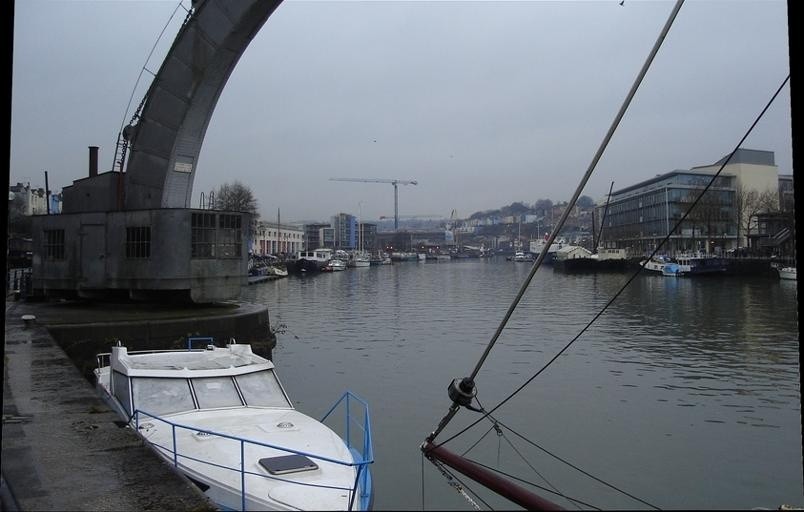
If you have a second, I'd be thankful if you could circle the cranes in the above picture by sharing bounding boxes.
[328,177,418,230]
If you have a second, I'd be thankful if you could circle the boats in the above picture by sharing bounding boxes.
[249,253,288,276]
[639,257,697,277]
[506,250,534,262]
[777,262,797,280]
[295,249,391,272]
[92,335,375,512]
